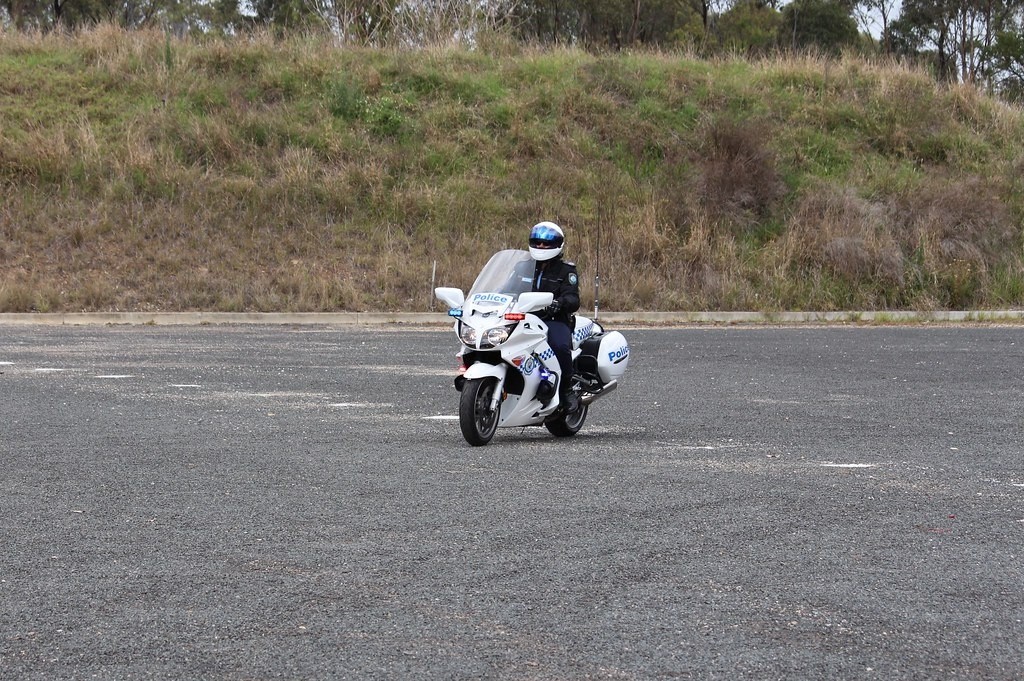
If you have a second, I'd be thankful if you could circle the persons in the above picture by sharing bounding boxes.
[499,221,583,416]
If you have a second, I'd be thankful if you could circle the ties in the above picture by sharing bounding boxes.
[536,263,549,290]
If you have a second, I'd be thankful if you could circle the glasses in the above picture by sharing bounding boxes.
[533,239,556,246]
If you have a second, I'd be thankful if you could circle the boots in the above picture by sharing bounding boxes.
[559,375,578,415]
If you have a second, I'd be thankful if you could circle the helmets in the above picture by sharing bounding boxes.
[528,221,565,261]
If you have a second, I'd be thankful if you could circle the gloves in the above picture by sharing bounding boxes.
[542,299,561,319]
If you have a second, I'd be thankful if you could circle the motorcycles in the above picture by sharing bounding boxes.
[434,201,630,446]
